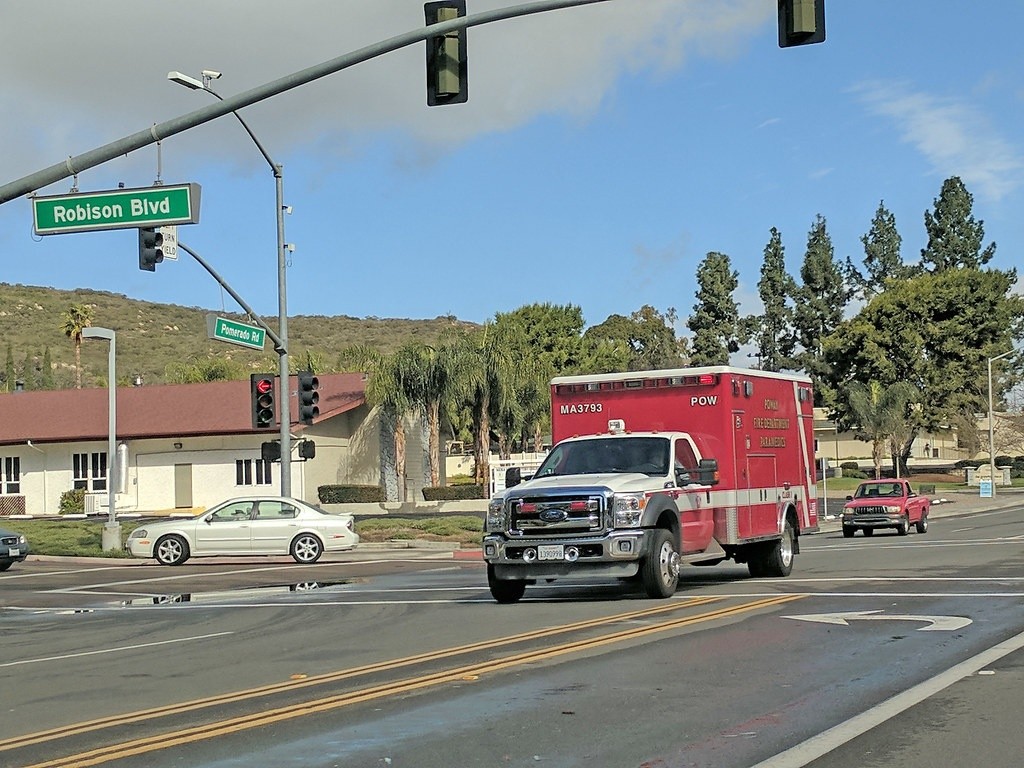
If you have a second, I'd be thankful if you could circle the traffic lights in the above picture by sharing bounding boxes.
[138,225,164,273]
[250,373,276,430]
[423,0,469,107]
[778,0,826,49]
[298,370,320,426]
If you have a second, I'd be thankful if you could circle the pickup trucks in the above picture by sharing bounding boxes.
[842,478,930,539]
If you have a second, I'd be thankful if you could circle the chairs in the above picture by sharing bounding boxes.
[869,489,878,498]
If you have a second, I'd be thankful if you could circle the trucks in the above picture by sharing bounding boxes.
[481,366,820,602]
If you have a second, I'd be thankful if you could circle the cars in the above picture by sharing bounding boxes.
[124,496,360,566]
[0,528,30,571]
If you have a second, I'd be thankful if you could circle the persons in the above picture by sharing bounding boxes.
[924,444,930,458]
[890,483,902,495]
[647,445,691,483]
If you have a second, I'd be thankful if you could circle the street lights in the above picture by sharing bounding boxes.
[988,343,1024,499]
[81,324,123,552]
